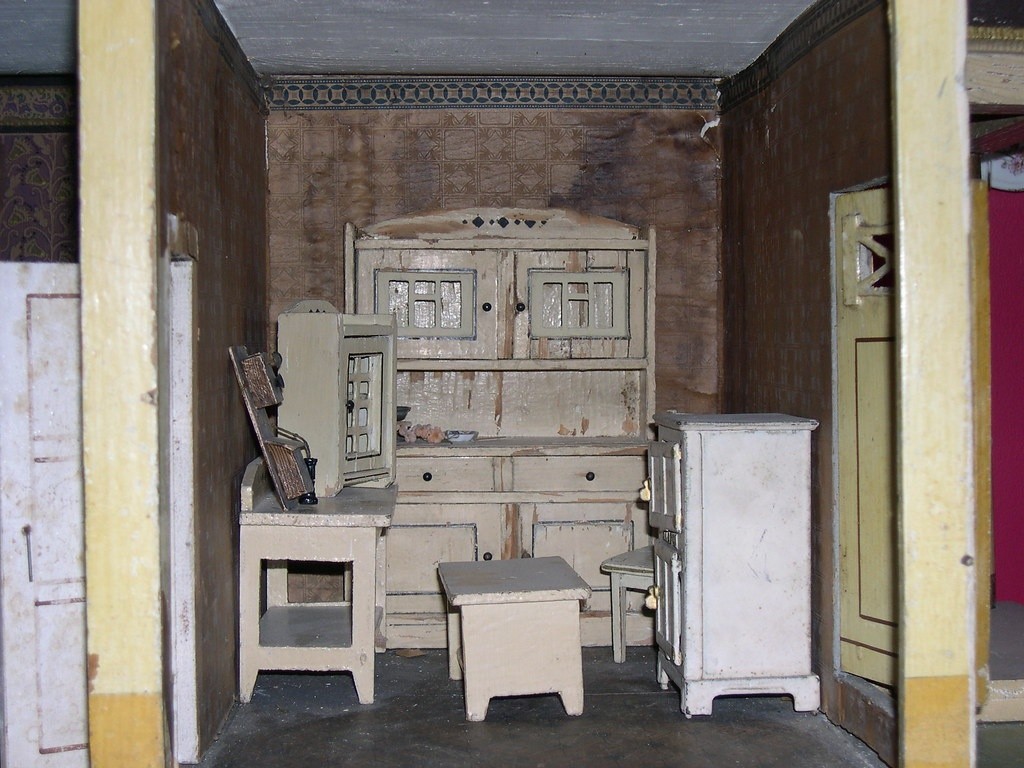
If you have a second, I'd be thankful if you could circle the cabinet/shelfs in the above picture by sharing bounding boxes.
[342,207,655,649]
[277,299,397,498]
[646,412,821,720]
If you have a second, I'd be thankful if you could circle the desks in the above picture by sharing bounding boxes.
[238,456,398,703]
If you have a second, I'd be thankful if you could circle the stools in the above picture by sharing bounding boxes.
[601,545,655,664]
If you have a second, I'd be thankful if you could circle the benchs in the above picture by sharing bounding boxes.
[437,555,591,721]
